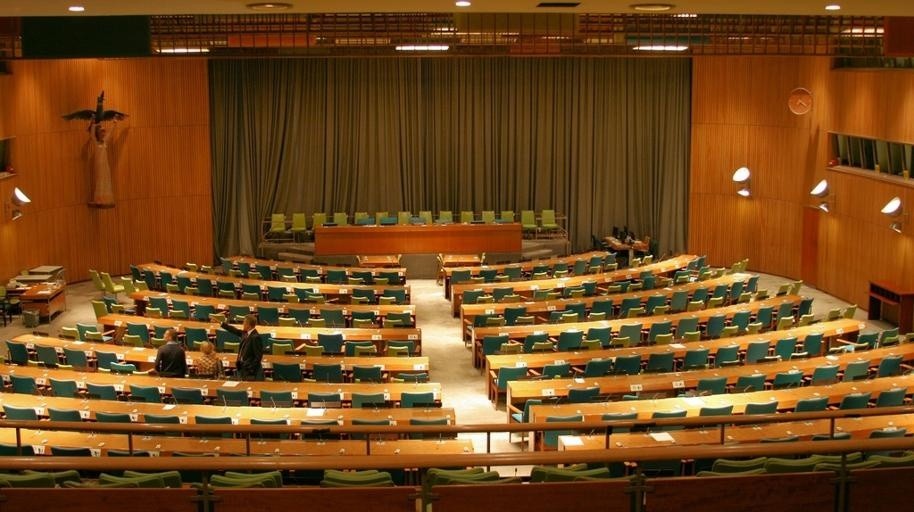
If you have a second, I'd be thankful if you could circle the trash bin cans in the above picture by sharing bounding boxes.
[23,310,40,327]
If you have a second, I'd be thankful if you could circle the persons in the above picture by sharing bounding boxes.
[86,117,118,205]
[208,314,263,381]
[154,329,186,377]
[191,339,226,379]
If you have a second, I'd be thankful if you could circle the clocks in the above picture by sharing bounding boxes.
[787,87,813,116]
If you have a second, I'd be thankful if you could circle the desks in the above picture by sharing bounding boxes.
[315,222,523,255]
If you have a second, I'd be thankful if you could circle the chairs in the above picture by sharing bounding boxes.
[436,252,913,468]
[60,481,140,488]
[0,473,56,488]
[591,225,658,267]
[1,481,13,488]
[531,467,645,512]
[20,470,83,484]
[424,469,523,504]
[190,470,283,512]
[0,254,474,457]
[697,451,912,476]
[98,473,166,488]
[269,210,559,240]
[123,471,183,488]
[320,468,396,487]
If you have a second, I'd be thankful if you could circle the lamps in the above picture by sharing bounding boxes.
[732,166,752,198]
[809,177,831,214]
[878,195,908,235]
[9,186,32,221]
